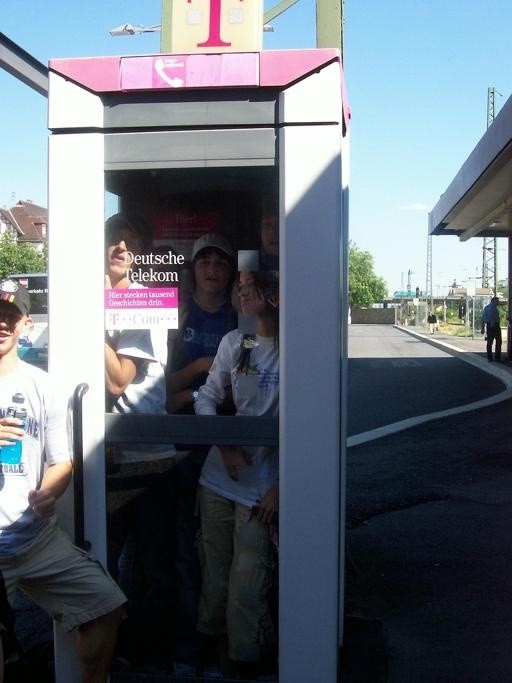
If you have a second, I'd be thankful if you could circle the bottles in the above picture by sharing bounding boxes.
[0,392,28,464]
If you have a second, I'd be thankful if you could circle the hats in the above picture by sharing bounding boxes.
[189,231,237,264]
[0,276,33,317]
[105,208,155,259]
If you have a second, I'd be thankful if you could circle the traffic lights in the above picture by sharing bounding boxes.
[458,302,466,320]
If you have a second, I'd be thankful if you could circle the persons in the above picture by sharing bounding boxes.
[427,311,437,334]
[0,276,127,683]
[479,296,502,362]
